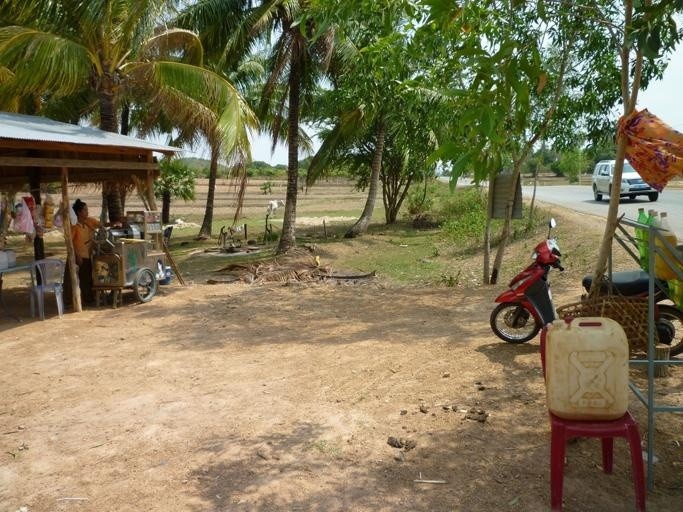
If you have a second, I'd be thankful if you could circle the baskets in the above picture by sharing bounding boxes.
[556,295,660,355]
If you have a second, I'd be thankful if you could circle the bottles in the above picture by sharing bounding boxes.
[636,207,683,308]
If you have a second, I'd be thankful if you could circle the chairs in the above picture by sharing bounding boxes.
[540,316,645,511]
[29,258,65,320]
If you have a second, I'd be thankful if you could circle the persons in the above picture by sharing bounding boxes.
[61,197,123,308]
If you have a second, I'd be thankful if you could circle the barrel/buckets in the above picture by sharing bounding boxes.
[545,318,629,419]
[160,266,172,285]
[5,248,17,268]
[0,248,8,269]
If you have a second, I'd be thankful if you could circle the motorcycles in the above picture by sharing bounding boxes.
[490,217,683,357]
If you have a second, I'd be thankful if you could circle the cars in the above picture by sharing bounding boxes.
[592,159,658,204]
